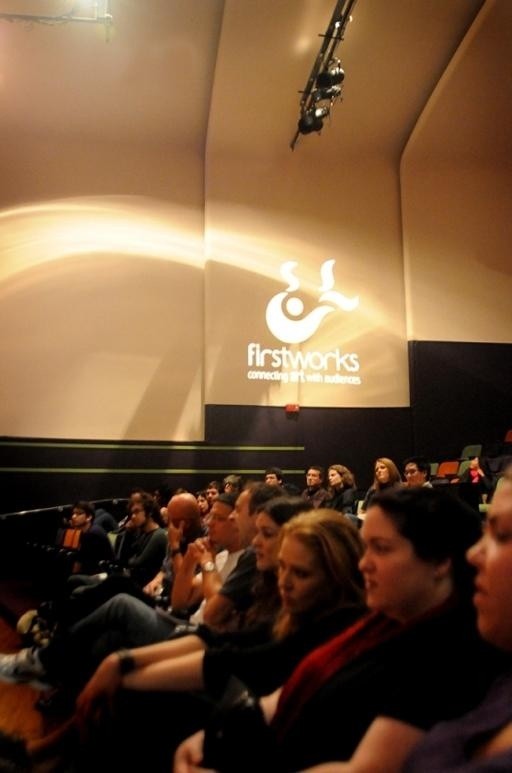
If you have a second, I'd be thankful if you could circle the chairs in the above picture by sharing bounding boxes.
[341,426,512,537]
[18,509,171,613]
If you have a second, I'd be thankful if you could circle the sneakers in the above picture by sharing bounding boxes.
[0,731,40,771]
[0,646,58,692]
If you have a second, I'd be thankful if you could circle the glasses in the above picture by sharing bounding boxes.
[72,511,85,515]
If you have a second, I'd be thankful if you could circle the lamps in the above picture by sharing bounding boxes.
[286,0,361,153]
